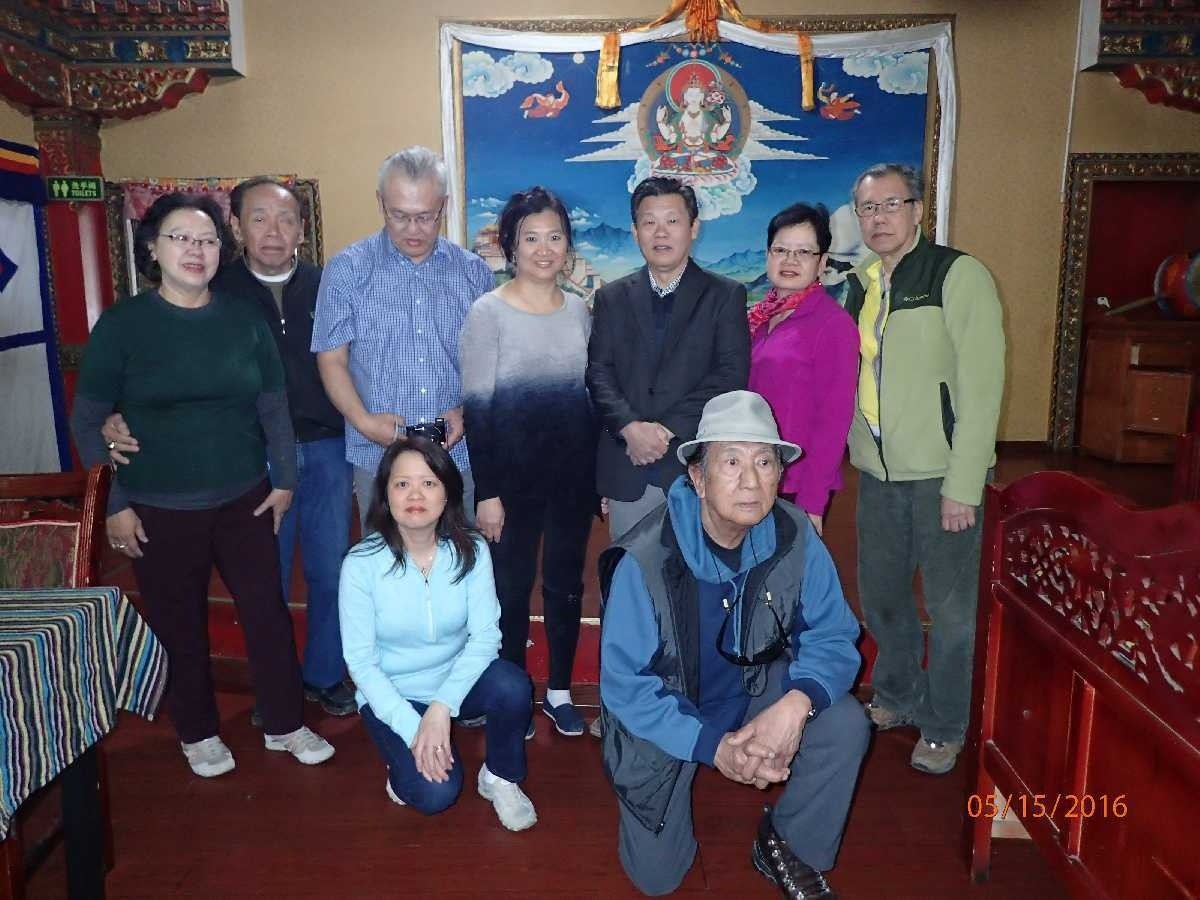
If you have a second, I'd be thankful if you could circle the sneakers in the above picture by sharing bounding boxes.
[304,680,358,716]
[542,693,584,736]
[264,725,335,764]
[251,703,264,727]
[477,762,538,832]
[589,716,602,738]
[909,730,966,773]
[385,762,410,806]
[181,735,236,778]
[861,703,918,731]
[524,718,535,740]
[751,806,834,900]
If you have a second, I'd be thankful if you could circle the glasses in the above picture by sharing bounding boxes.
[161,232,223,250]
[854,198,918,218]
[716,595,788,666]
[768,247,821,260]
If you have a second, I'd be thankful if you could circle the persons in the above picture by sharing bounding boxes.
[597,390,872,900]
[72,191,338,776]
[309,145,478,542]
[337,433,540,832]
[585,177,753,539]
[460,185,611,739]
[102,179,358,717]
[847,162,1008,774]
[747,204,860,535]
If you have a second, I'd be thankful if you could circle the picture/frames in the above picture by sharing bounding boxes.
[440,14,956,309]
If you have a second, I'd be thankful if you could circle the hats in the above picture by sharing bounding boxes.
[677,389,802,467]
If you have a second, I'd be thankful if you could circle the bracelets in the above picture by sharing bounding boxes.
[807,709,815,718]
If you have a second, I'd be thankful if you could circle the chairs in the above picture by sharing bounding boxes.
[0,465,116,875]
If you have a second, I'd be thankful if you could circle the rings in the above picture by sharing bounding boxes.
[119,544,127,549]
[434,745,444,753]
[107,441,115,450]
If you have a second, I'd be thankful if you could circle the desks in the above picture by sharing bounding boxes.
[0,586,169,900]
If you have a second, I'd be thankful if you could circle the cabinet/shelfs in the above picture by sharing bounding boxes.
[1080,322,1200,461]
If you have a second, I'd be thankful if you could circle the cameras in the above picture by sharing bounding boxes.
[407,418,446,445]
